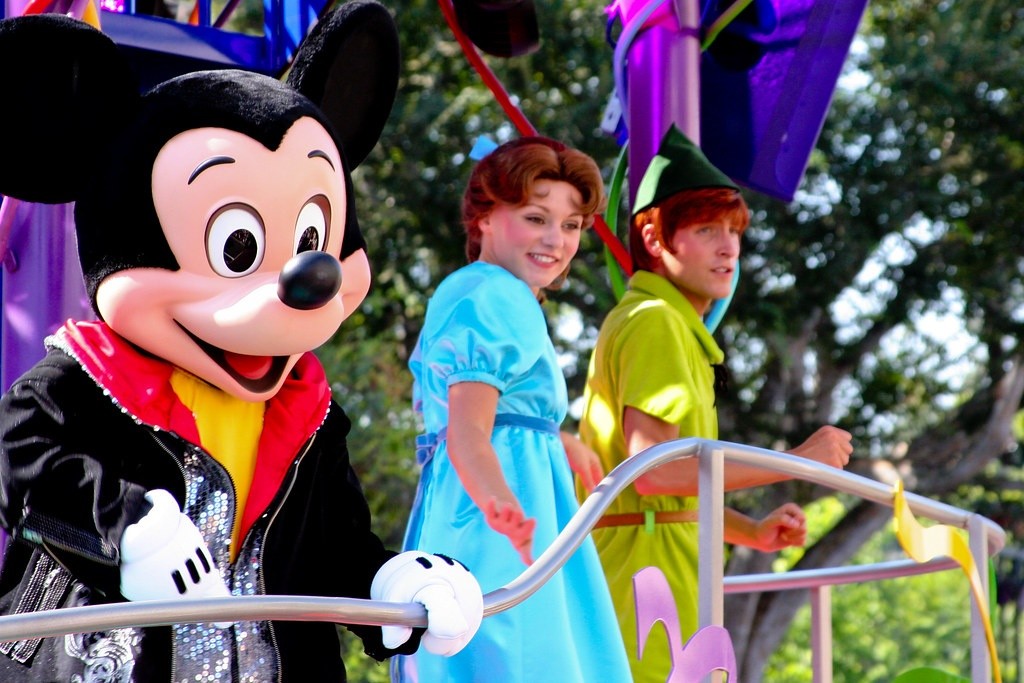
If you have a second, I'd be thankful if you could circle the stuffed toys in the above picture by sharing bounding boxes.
[1,0,484,681]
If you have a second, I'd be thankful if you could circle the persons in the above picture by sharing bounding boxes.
[387,136,633,682]
[575,122,853,683]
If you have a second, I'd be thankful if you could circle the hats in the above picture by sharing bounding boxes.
[632,120,741,216]
